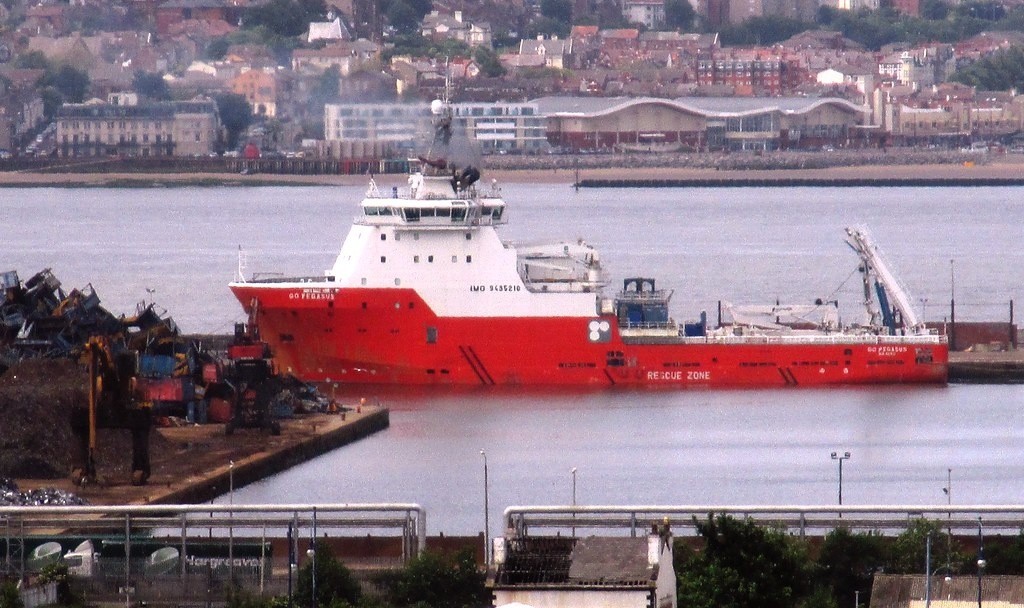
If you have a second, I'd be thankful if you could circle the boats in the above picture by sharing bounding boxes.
[225,55,950,385]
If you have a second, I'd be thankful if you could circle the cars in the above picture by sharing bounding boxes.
[187,151,305,158]
[25,122,58,157]
[482,146,606,155]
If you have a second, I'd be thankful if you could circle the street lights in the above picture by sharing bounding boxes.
[306,505,317,608]
[572,467,578,537]
[479,447,489,571]
[228,459,235,582]
[976,517,987,608]
[831,451,850,518]
